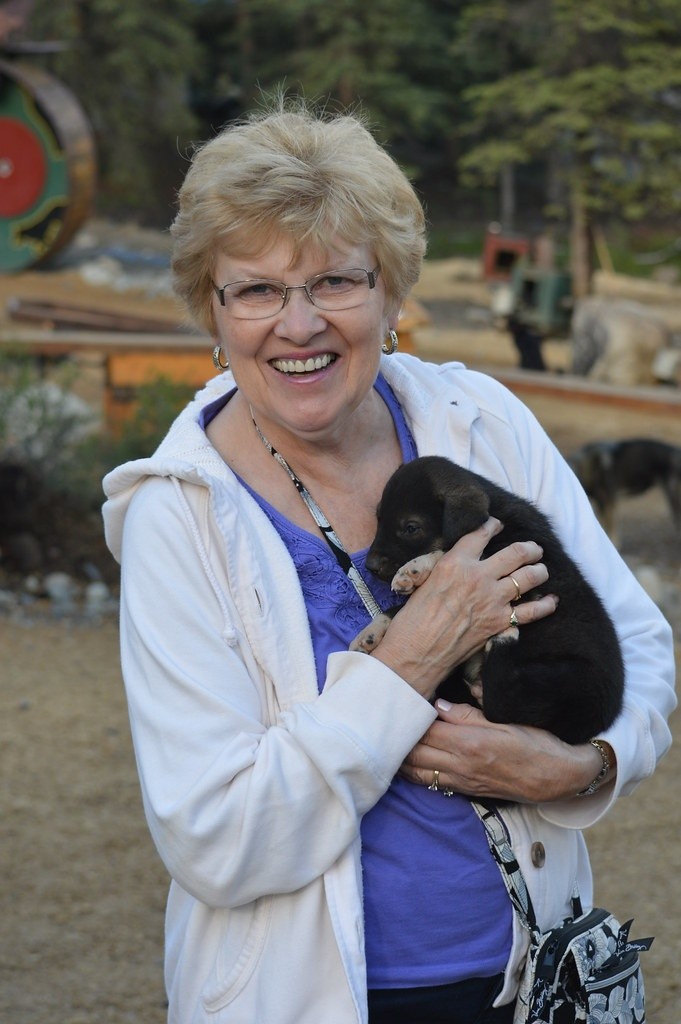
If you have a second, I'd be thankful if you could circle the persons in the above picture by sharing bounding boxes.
[101,108,676,1024]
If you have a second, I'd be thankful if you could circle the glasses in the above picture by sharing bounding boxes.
[211,262,381,320]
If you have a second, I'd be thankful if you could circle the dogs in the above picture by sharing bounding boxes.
[346,454,625,813]
[563,439,681,552]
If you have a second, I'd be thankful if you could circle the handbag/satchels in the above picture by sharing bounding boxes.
[513,909,655,1024]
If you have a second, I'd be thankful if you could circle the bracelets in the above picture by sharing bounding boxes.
[577,739,611,797]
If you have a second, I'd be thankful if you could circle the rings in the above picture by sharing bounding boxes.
[442,783,454,797]
[510,607,519,630]
[427,770,440,792]
[507,574,521,603]
